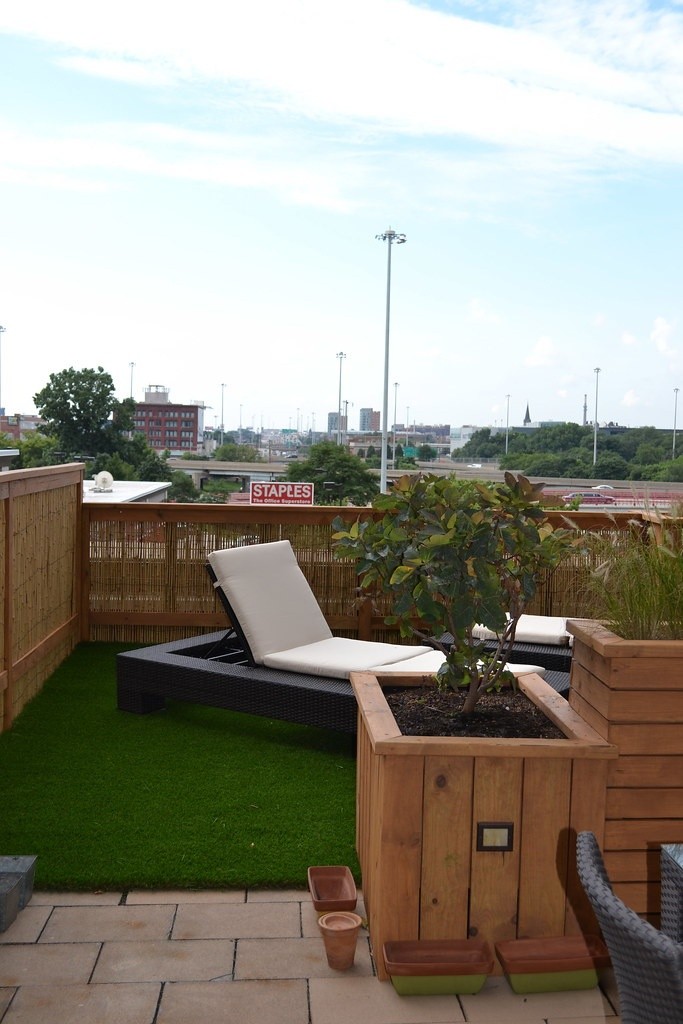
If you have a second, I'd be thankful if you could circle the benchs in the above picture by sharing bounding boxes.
[469,609,607,649]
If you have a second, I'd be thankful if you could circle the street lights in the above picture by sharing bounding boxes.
[129,361,135,403]
[220,383,225,446]
[593,368,601,464]
[337,352,347,447]
[375,225,407,495]
[392,382,398,469]
[671,388,679,461]
[506,394,511,454]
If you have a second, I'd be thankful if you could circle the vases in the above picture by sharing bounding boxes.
[308,866,358,919]
[494,935,614,994]
[382,938,494,996]
[318,911,363,969]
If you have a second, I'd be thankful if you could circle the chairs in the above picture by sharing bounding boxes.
[575,829,683,1024]
[202,539,548,694]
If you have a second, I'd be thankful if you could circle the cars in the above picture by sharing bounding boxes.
[562,492,616,505]
[286,454,297,458]
[592,485,614,490]
[467,464,482,468]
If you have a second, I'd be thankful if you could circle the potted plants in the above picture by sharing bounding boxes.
[325,469,620,984]
[557,480,683,936]
